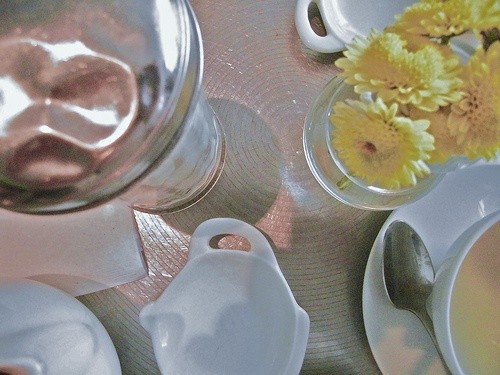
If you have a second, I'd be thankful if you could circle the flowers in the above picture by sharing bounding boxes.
[329,0,500,192]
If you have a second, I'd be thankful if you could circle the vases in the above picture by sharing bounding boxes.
[303,73,454,211]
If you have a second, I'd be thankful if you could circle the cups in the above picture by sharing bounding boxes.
[0,0,226,214]
[302,73,463,212]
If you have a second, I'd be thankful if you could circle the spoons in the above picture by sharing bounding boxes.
[382,219,453,375]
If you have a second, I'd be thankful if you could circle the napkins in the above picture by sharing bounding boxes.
[0,200,149,298]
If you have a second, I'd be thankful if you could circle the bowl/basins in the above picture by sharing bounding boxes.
[432,210,500,375]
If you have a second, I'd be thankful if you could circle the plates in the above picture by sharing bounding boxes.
[361,156,500,375]
[0,278,122,375]
[139,217,310,375]
[295,0,422,53]
